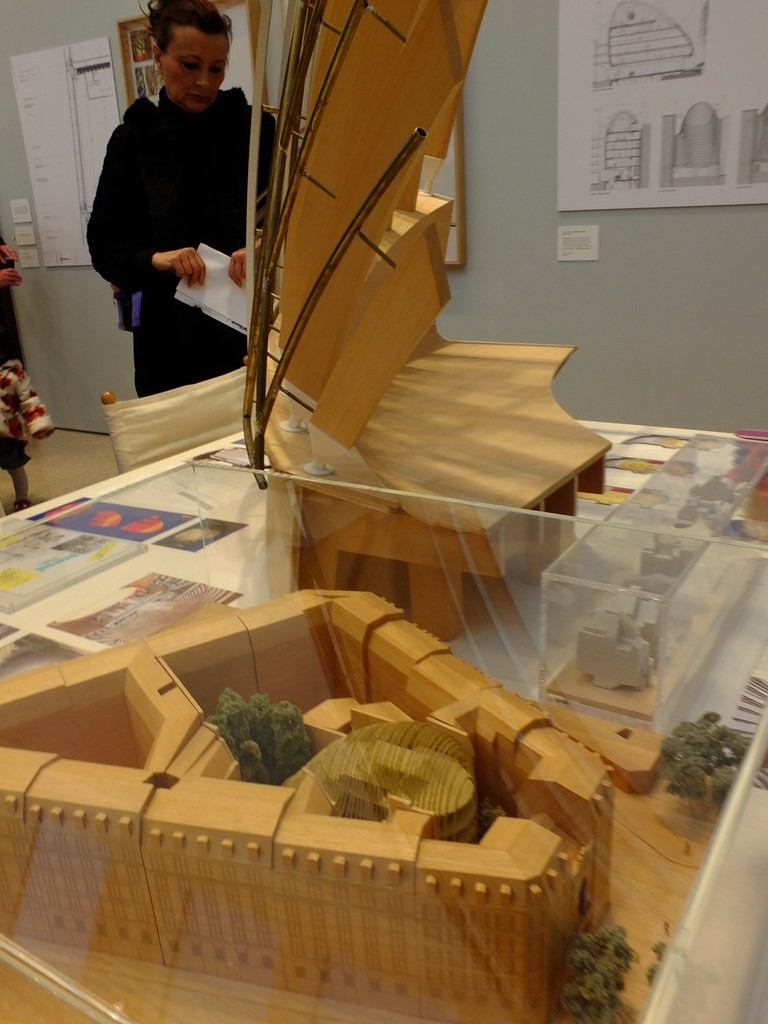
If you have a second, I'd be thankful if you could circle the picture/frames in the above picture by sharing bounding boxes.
[118,15,165,107]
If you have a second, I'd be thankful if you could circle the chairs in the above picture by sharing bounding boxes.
[102,357,251,473]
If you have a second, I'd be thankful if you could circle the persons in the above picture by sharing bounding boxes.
[0,235,54,513]
[86,1,278,397]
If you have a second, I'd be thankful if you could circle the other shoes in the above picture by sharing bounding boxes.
[14,499,32,513]
